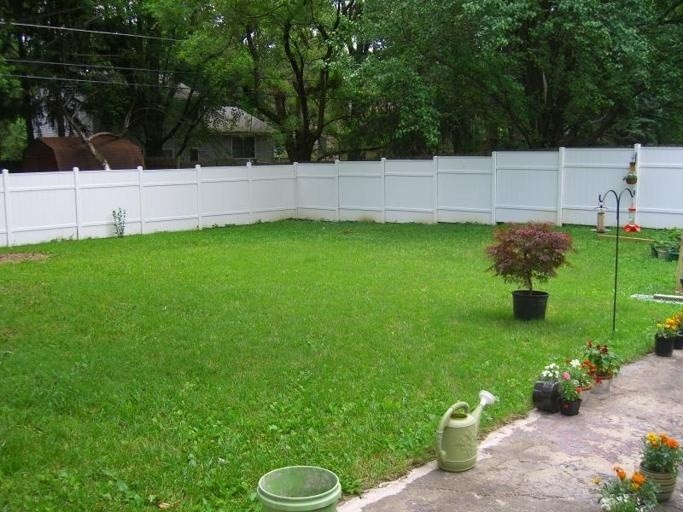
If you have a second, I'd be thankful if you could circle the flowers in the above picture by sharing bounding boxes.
[637,429,681,467]
[540,358,590,400]
[591,462,655,512]
[653,318,674,336]
[581,339,620,377]
[672,314,682,335]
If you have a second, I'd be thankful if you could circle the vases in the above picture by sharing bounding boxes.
[636,464,678,505]
[561,398,584,418]
[532,380,557,415]
[653,333,674,356]
[590,371,613,394]
[672,331,682,350]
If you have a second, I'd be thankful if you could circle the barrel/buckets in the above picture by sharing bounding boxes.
[255,465,341,510]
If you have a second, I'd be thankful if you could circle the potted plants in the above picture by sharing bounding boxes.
[483,215,576,320]
[650,223,681,263]
